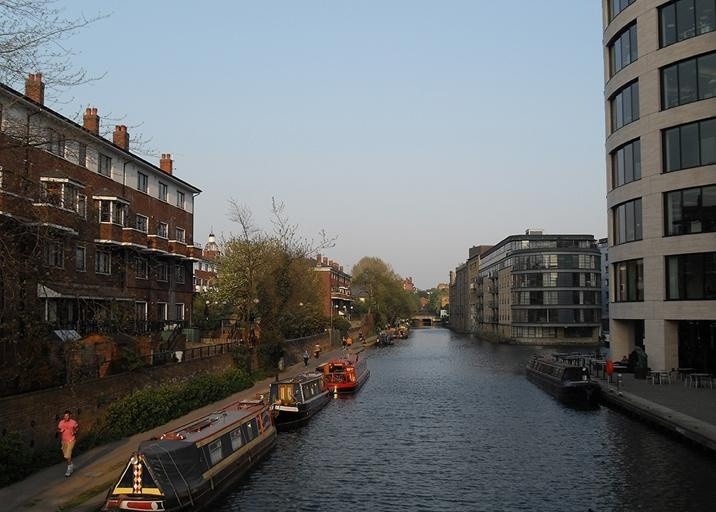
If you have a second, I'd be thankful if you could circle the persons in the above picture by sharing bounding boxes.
[342,335,347,349]
[55,410,79,477]
[314,342,322,359]
[302,348,309,369]
[346,336,352,350]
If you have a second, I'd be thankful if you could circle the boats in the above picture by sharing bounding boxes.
[526,354,602,407]
[101,394,279,512]
[267,369,332,430]
[376,318,412,347]
[322,352,371,395]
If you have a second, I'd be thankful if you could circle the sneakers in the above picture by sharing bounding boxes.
[65,464,73,476]
